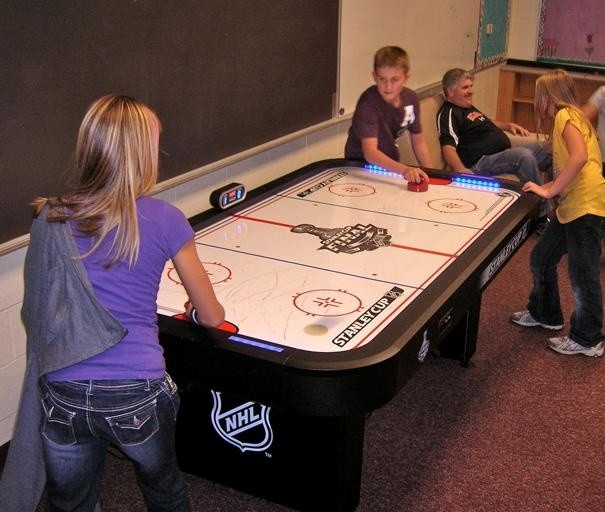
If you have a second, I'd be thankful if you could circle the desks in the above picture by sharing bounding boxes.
[121,158,546,511]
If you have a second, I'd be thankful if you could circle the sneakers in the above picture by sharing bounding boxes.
[535,218,550,235]
[511,310,563,330]
[546,335,604,358]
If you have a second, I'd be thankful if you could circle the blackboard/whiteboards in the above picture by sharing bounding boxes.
[0,1,342,257]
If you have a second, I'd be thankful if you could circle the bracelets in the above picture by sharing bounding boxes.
[190,306,199,326]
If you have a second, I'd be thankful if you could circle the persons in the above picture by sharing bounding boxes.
[583,84,605,174]
[344,46,434,184]
[436,68,552,235]
[511,70,605,357]
[0,94,225,512]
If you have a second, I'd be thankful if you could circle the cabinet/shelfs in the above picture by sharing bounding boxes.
[495,63,605,136]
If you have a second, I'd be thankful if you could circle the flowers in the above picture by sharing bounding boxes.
[581,32,595,62]
[539,35,559,59]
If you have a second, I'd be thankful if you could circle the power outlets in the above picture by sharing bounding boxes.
[486,24,494,34]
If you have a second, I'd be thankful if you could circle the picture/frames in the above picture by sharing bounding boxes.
[473,0,511,73]
[536,1,604,68]
